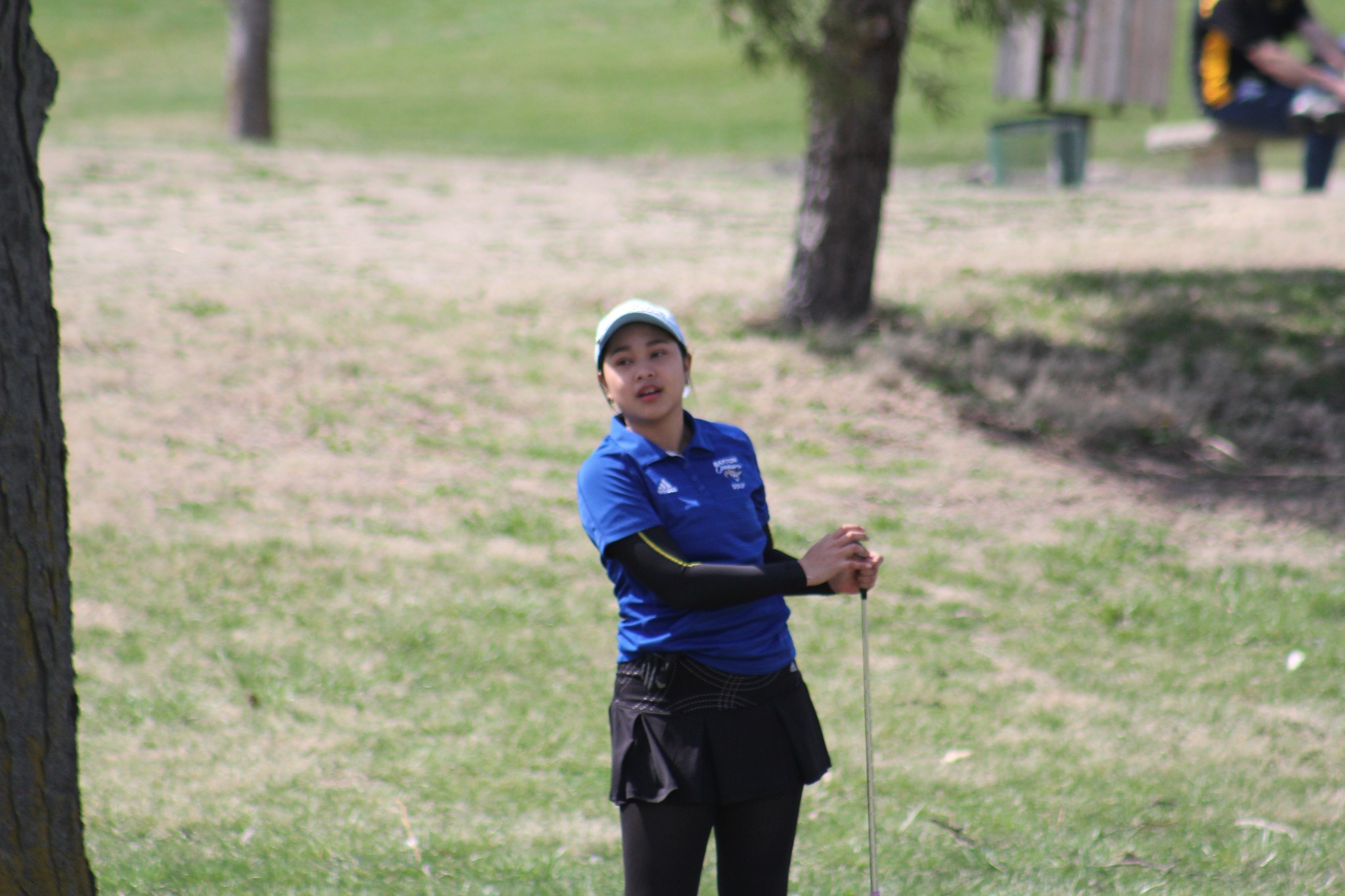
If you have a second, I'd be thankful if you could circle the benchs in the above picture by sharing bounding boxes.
[1149,120,1303,187]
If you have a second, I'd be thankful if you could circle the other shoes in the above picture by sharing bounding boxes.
[1287,88,1345,125]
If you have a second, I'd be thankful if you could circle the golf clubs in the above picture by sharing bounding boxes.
[855,590,882,896]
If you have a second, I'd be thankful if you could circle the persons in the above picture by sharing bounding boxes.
[577,301,884,896]
[1193,0,1345,192]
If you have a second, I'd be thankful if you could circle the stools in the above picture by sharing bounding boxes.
[989,113,1088,182]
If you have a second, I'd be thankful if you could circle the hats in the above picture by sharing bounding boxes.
[594,297,687,372]
